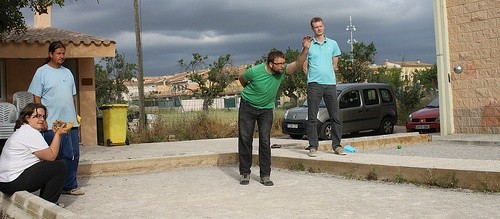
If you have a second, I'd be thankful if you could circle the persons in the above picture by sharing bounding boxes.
[302,17,347,156]
[0,103,65,208]
[27,41,84,195]
[237,34,314,186]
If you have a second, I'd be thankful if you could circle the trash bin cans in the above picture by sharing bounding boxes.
[99,103,130,147]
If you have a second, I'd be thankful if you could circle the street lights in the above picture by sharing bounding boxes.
[346,15,359,65]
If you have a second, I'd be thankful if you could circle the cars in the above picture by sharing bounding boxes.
[405,96,440,134]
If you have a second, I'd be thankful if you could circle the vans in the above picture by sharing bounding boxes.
[282,82,398,139]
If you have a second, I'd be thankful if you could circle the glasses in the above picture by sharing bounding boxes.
[31,114,46,120]
[272,62,286,66]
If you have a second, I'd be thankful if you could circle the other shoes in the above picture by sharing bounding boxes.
[334,146,347,155]
[240,174,249,185]
[261,176,274,186]
[61,188,84,194]
[309,149,317,156]
[56,203,64,208]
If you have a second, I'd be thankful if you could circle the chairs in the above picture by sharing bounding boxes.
[0,91,34,139]
